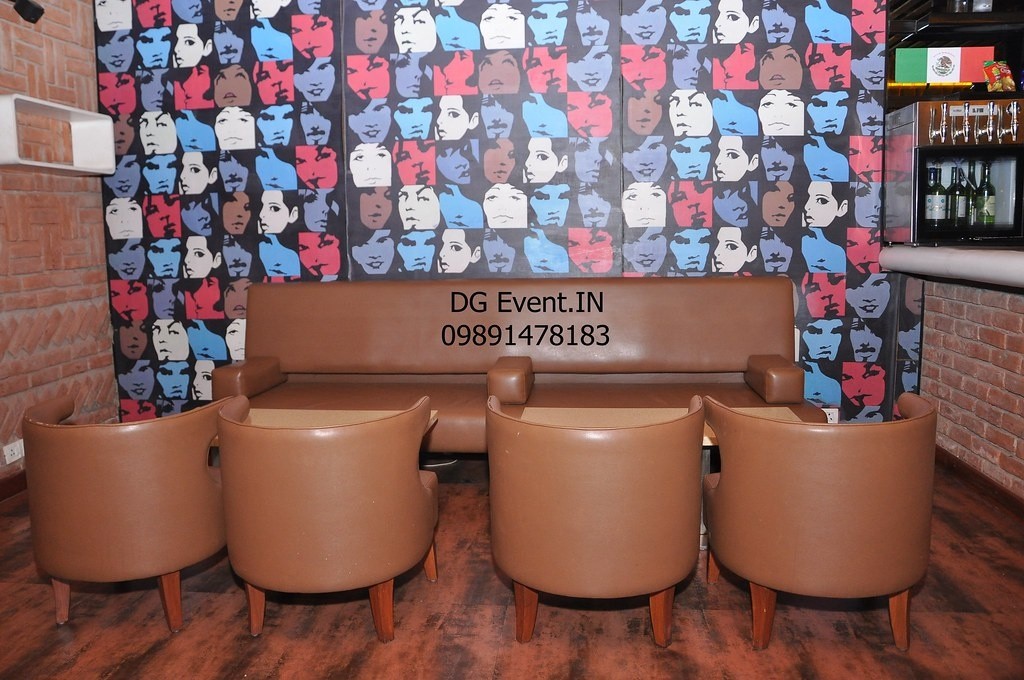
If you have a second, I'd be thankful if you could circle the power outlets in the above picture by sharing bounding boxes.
[821,408,840,423]
[4,439,25,465]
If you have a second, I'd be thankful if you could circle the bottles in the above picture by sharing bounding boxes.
[926,160,977,226]
[976,163,996,225]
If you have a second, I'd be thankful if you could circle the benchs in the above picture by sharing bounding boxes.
[211,276,827,452]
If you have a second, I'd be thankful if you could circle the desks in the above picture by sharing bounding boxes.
[210,410,440,447]
[519,405,807,550]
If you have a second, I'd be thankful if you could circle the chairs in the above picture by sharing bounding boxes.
[218,396,440,643]
[486,393,706,647]
[23,394,251,633]
[702,392,937,652]
